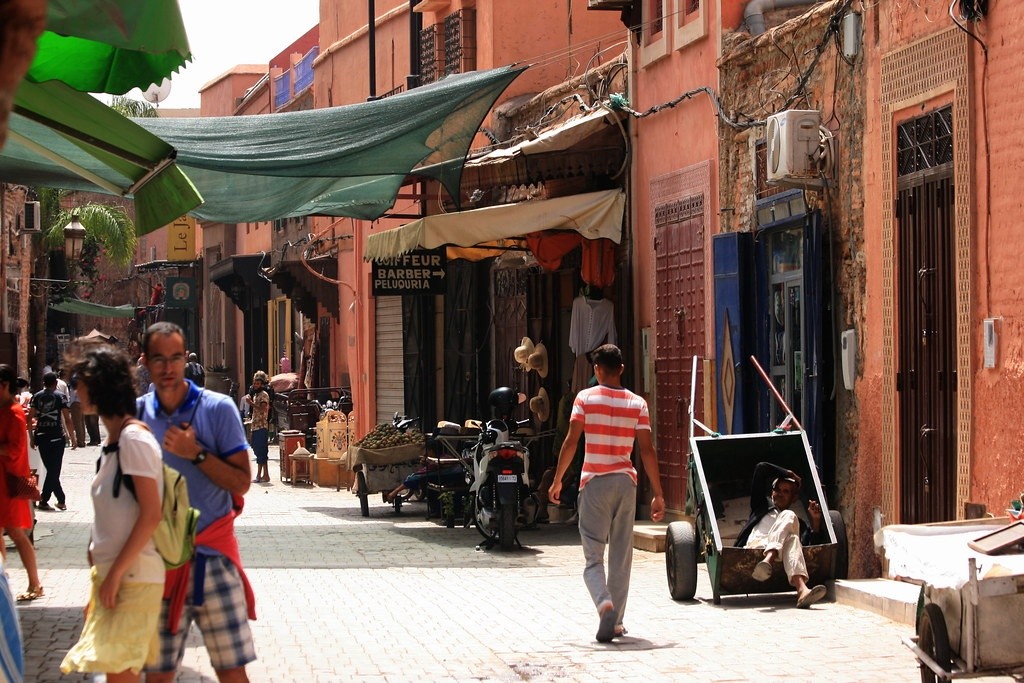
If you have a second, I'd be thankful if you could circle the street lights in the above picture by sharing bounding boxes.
[63,213,87,335]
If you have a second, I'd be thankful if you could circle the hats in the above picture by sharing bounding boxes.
[528,343,548,378]
[513,337,534,372]
[529,386,549,422]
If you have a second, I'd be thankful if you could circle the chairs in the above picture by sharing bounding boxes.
[327,459,351,492]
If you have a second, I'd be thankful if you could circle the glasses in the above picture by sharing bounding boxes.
[146,354,186,371]
[774,487,791,496]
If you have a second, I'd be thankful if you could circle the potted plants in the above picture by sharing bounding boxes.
[205,363,231,395]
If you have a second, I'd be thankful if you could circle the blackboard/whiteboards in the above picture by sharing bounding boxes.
[369,246,449,296]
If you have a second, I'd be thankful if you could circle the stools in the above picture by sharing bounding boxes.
[288,454,314,486]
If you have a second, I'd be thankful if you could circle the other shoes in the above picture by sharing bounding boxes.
[38,504,55,511]
[752,561,772,581]
[253,476,270,483]
[797,585,826,609]
[55,502,66,509]
[87,442,98,446]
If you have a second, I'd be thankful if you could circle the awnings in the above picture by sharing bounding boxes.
[134,259,200,289]
[48,296,133,318]
[308,149,489,218]
[363,186,627,264]
[0,73,204,237]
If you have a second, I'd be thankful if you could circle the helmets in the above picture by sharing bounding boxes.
[489,387,519,408]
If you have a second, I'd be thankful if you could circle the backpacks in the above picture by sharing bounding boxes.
[112,417,201,570]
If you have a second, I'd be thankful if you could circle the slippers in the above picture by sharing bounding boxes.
[596,606,618,643]
[615,626,628,638]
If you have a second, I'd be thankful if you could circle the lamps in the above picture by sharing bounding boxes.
[27,214,87,283]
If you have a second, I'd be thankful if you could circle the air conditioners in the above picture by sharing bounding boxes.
[766,110,821,180]
[23,201,41,231]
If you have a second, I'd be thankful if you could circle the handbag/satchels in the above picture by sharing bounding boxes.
[8,474,41,501]
[33,428,39,445]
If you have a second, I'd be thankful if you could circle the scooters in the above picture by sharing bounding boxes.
[468,388,530,552]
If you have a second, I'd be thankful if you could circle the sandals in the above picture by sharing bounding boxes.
[17,586,45,599]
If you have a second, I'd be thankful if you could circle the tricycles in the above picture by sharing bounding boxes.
[353,412,426,515]
[273,385,353,450]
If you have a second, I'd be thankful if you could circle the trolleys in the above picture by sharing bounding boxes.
[902,558,1024,683]
[664,353,848,606]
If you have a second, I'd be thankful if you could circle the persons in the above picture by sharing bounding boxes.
[59,348,166,683]
[735,462,825,609]
[0,364,44,603]
[136,357,152,395]
[387,443,462,503]
[240,371,270,483]
[548,344,664,643]
[27,366,101,511]
[135,322,258,683]
[185,352,204,387]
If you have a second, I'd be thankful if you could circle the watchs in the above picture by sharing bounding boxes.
[192,449,208,465]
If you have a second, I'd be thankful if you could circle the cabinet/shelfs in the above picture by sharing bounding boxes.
[424,432,479,527]
[278,432,306,485]
